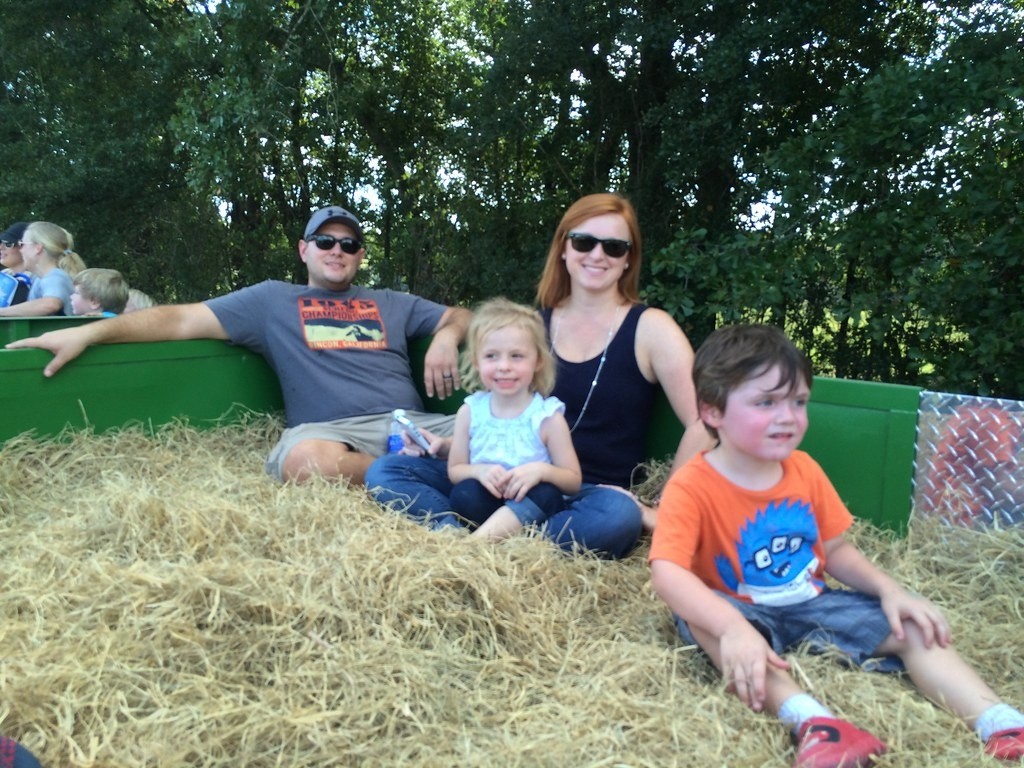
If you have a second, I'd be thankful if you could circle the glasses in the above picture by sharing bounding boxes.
[306,234,361,254]
[0,240,17,249]
[568,232,633,257]
[18,240,31,247]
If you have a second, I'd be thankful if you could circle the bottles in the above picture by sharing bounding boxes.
[387,409,406,454]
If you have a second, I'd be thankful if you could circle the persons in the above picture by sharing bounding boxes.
[647,325,1024,768]
[6,207,477,485]
[447,295,582,543]
[363,192,719,561]
[0,221,155,317]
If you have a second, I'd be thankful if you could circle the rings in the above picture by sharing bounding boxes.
[443,375,452,378]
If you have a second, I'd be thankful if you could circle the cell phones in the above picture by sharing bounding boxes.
[395,416,438,459]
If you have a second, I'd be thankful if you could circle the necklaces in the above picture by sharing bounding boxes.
[549,305,620,435]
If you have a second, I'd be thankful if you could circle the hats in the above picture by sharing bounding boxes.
[304,206,359,241]
[0,222,29,241]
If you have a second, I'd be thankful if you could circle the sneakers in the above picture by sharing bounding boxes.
[791,716,886,768]
[984,726,1024,760]
